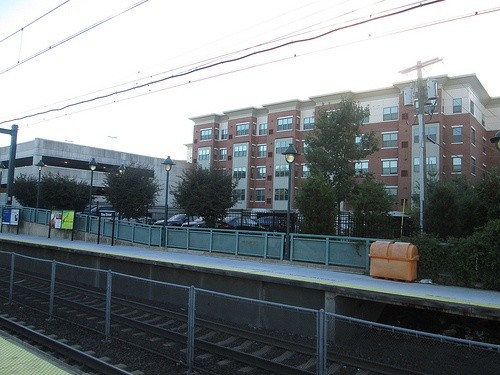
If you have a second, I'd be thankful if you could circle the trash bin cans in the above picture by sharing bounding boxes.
[367,241,419,282]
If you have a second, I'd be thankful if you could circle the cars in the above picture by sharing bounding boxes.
[83,205,348,237]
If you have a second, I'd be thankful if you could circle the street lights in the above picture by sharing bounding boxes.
[161,156,176,248]
[280,143,301,260]
[34,159,46,223]
[86,157,100,233]
